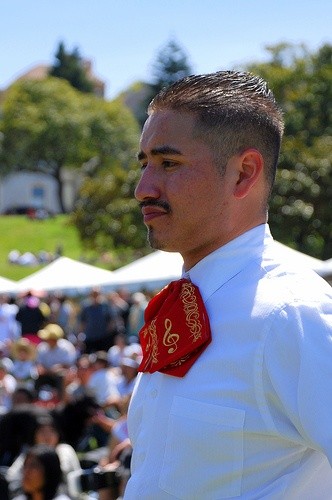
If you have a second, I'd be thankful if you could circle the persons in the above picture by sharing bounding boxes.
[0,291,158,500]
[123,69,332,499]
[3,203,55,222]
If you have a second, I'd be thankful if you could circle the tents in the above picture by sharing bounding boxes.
[0,242,185,300]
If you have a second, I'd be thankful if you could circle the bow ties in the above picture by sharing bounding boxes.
[140,279,210,373]
